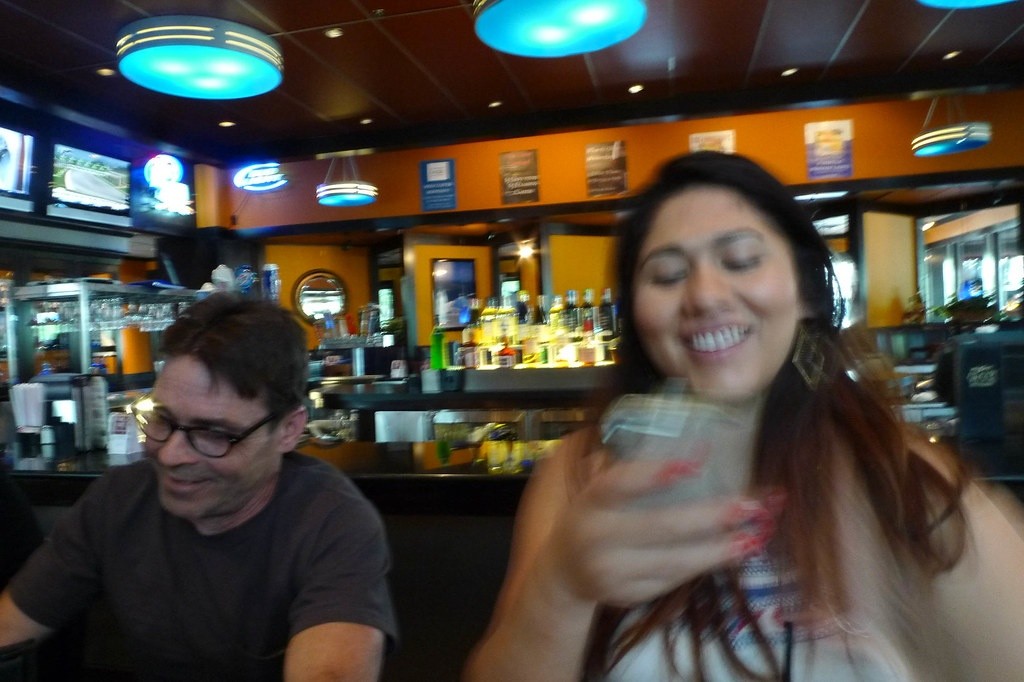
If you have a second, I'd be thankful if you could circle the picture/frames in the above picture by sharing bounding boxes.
[430,258,479,331]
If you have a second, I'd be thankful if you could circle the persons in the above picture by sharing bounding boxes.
[468,150,1024,682]
[0,290,388,682]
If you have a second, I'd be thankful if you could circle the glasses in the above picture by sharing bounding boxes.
[129,390,288,458]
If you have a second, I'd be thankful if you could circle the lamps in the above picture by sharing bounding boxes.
[116,15,285,100]
[910,51,992,156]
[472,0,649,59]
[325,25,343,38]
[315,148,379,206]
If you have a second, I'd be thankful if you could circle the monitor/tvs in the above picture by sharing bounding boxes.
[0,121,41,217]
[938,333,1024,446]
[44,139,134,229]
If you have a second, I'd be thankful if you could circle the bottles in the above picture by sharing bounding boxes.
[425,286,621,370]
[311,288,397,350]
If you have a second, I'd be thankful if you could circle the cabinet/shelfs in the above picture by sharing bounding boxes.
[869,322,952,438]
[0,281,194,374]
